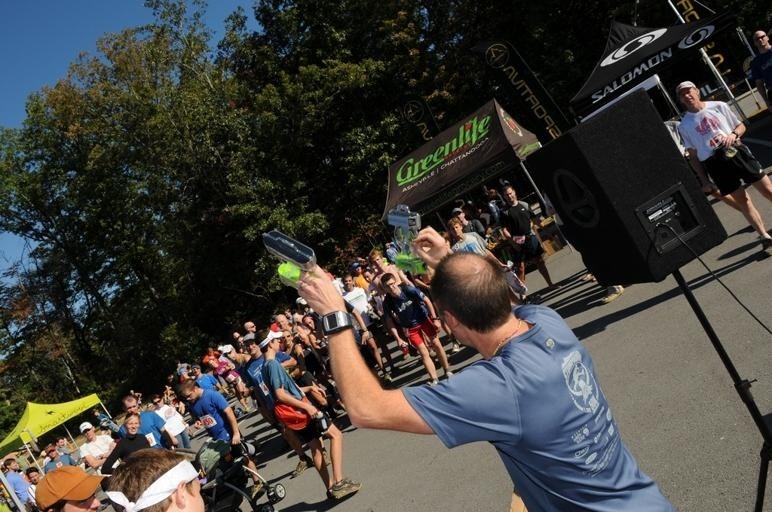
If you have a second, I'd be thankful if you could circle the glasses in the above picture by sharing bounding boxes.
[153,398,161,405]
[282,335,291,339]
[184,392,193,399]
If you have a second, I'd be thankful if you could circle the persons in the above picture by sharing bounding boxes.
[233,332,240,340]
[295,225,674,512]
[237,336,248,357]
[676,81,772,251]
[76,422,116,473]
[178,378,263,497]
[284,309,293,320]
[446,219,525,309]
[499,184,563,293]
[119,394,179,451]
[98,412,151,475]
[4,458,29,511]
[362,268,420,361]
[280,328,336,424]
[106,448,205,512]
[381,271,452,385]
[293,311,302,326]
[244,321,257,335]
[368,248,440,362]
[749,29,772,109]
[152,395,192,450]
[489,188,505,212]
[202,347,221,364]
[43,444,80,473]
[301,316,334,376]
[191,364,221,392]
[452,208,488,243]
[351,262,371,294]
[255,328,363,503]
[25,467,43,512]
[221,344,251,376]
[55,437,78,457]
[173,359,192,375]
[241,333,332,480]
[275,315,338,404]
[384,243,398,263]
[339,273,399,376]
[207,358,254,415]
[91,410,120,434]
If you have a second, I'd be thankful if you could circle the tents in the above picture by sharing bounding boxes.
[0,392,114,476]
[568,7,757,134]
[377,98,551,242]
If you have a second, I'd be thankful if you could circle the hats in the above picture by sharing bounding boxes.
[452,208,463,215]
[243,333,256,342]
[45,445,55,454]
[258,330,283,349]
[676,81,697,101]
[80,421,93,433]
[222,344,233,355]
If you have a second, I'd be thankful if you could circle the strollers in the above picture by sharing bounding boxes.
[200,456,286,511]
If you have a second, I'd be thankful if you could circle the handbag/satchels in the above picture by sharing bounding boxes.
[504,261,528,295]
[268,358,309,431]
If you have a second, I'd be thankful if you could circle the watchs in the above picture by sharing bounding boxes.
[510,235,514,242]
[319,310,355,335]
[732,131,740,139]
[432,317,440,321]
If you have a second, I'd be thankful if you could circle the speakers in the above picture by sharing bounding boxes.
[521,87,729,289]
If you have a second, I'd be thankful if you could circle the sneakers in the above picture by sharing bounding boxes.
[602,289,624,303]
[323,450,331,466]
[292,458,313,477]
[432,377,439,385]
[333,479,362,498]
[549,284,561,289]
[445,371,453,377]
[384,372,392,383]
[582,273,593,280]
[326,490,334,500]
[251,483,263,499]
[762,235,772,254]
[591,277,596,282]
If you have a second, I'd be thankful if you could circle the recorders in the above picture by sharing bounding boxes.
[387,204,420,230]
[262,229,316,271]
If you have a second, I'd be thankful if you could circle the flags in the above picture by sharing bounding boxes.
[397,95,441,143]
[474,36,570,145]
[669,0,745,95]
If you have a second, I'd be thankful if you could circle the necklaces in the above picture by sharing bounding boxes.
[491,318,528,358]
